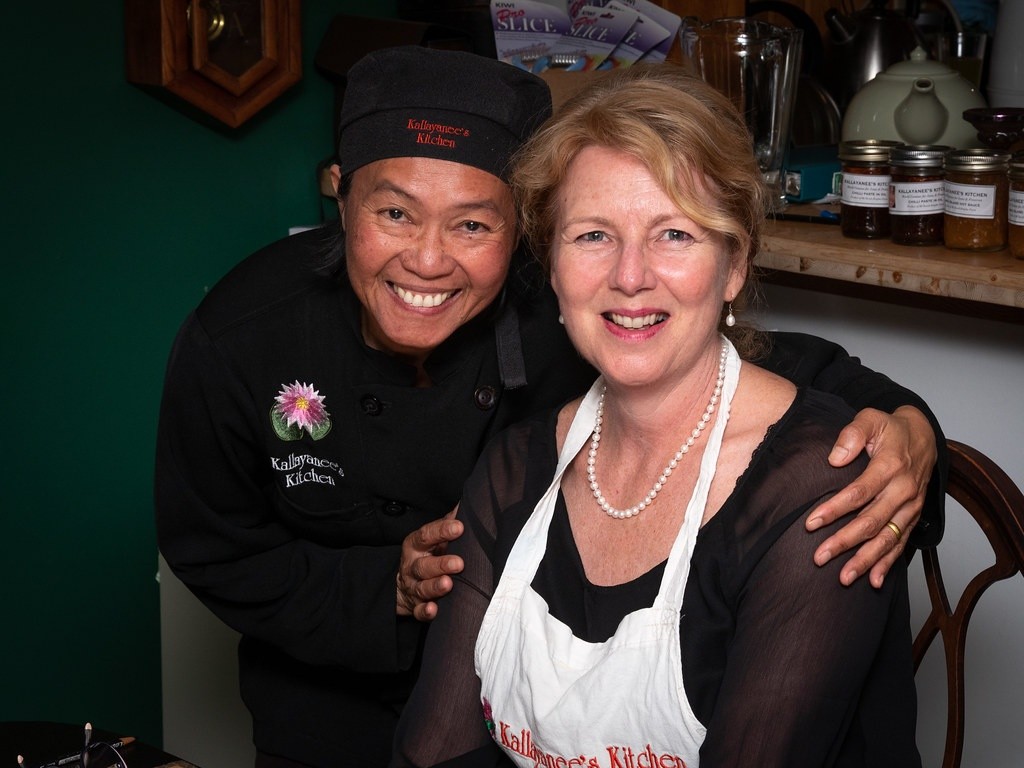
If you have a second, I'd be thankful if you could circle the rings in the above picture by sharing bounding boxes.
[884,521,901,545]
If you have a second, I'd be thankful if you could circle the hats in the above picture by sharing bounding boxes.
[339,45,553,184]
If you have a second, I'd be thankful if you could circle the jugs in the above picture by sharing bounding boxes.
[678,16,802,211]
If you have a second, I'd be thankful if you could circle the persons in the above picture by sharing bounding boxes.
[391,60,924,768]
[157,45,946,768]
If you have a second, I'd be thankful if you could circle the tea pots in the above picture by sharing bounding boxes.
[824,0,963,110]
[732,0,842,150]
[840,46,990,155]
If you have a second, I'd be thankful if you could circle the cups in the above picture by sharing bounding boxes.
[933,31,987,91]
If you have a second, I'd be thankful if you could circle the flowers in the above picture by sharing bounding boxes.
[271,380,332,443]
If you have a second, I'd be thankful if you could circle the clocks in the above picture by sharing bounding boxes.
[123,1,304,132]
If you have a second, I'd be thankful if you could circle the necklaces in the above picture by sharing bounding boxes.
[584,333,728,516]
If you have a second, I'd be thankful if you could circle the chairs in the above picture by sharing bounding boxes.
[910,439,1024,768]
